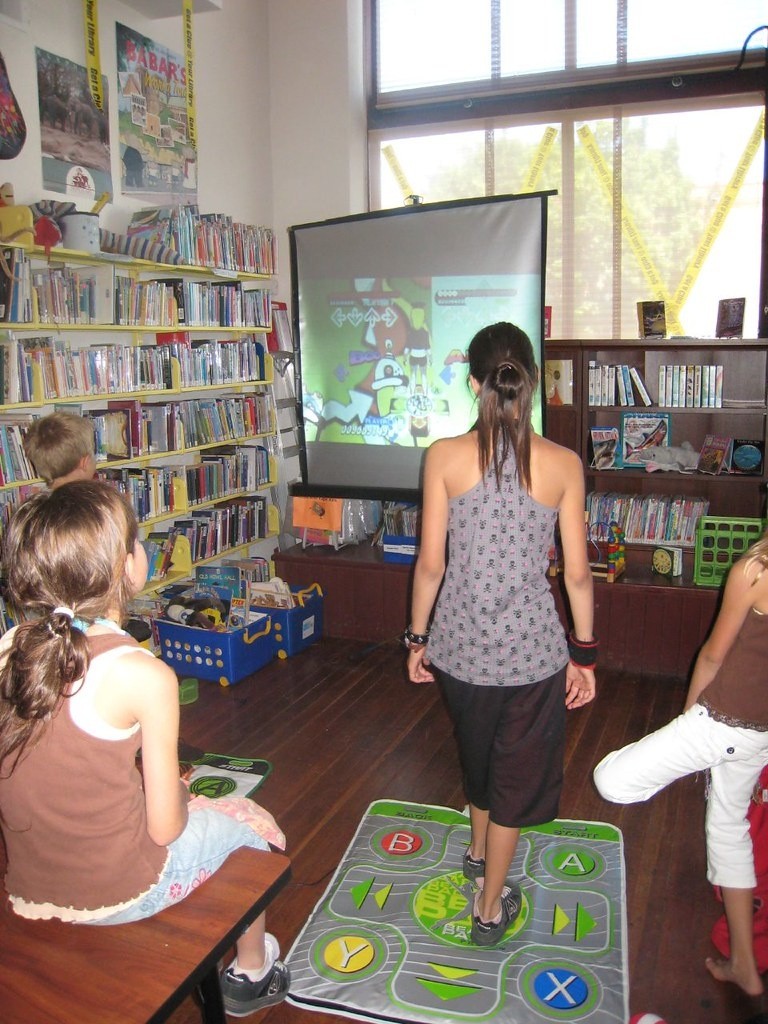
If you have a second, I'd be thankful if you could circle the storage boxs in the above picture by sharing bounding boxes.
[154,582,329,687]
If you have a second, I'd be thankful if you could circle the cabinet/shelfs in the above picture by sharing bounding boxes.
[270,339,768,677]
[0,240,281,600]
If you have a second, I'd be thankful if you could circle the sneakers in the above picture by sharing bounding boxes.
[221,956,291,1017]
[462,841,485,880]
[470,880,521,946]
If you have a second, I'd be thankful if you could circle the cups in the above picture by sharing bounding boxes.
[58,212,101,254]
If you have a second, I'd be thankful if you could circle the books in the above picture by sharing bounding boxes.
[129,203,278,275]
[0,329,142,634]
[590,425,624,471]
[695,435,763,476]
[589,361,766,409]
[136,332,297,652]
[585,491,710,546]
[715,298,745,336]
[637,300,666,337]
[620,411,672,469]
[384,504,423,538]
[0,246,271,328]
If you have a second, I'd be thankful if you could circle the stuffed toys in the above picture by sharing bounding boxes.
[638,441,701,472]
[163,591,227,629]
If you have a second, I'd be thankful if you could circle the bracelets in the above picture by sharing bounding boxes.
[403,626,431,651]
[569,630,599,669]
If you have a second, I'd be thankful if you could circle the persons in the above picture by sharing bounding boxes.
[25,413,147,632]
[0,480,289,1017]
[405,321,596,946]
[589,534,767,997]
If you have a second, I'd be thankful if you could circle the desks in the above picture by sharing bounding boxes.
[0,831,291,1024]
